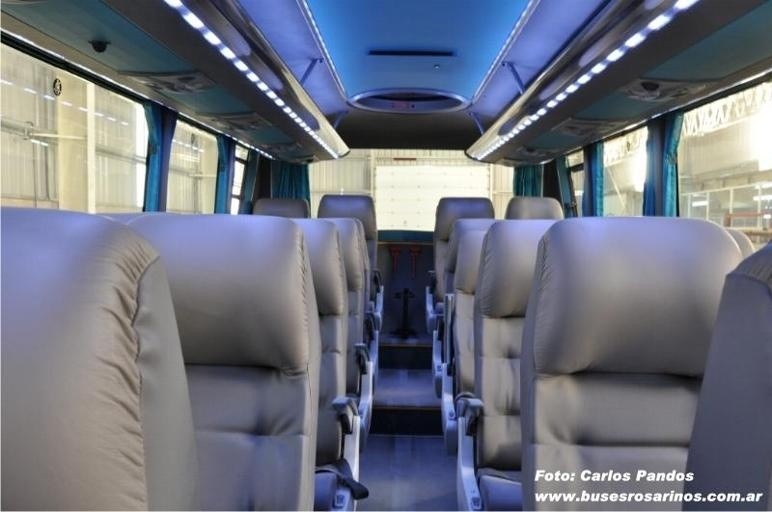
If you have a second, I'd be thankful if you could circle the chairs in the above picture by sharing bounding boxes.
[425,190,771,511]
[0,185,386,512]
